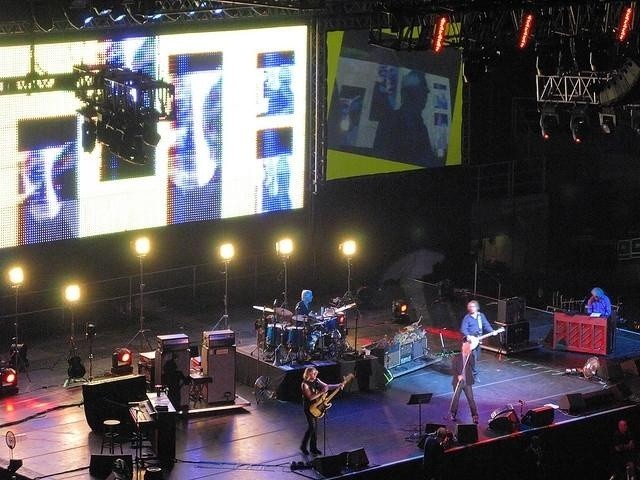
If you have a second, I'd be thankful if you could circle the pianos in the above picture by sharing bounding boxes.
[129,392,176,463]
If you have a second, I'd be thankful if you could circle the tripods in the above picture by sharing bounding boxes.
[50,302,88,385]
[211,260,229,330]
[279,257,293,310]
[5,283,33,385]
[342,257,359,304]
[250,303,359,367]
[122,256,157,352]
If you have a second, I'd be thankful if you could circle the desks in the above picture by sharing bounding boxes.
[190,373,213,407]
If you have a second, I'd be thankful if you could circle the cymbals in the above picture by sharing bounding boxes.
[292,315,309,322]
[335,302,356,312]
[253,306,293,316]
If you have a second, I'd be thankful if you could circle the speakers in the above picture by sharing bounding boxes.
[557,382,630,416]
[418,423,446,451]
[489,410,519,434]
[88,453,133,480]
[155,350,190,406]
[497,296,525,323]
[311,447,370,478]
[521,405,554,427]
[201,346,236,402]
[455,423,478,444]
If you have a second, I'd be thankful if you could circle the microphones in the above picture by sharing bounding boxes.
[314,378,326,387]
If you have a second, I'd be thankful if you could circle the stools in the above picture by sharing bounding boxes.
[99,418,127,458]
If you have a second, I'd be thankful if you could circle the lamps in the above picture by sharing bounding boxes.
[272,236,299,317]
[1,366,20,398]
[109,343,134,375]
[534,72,638,145]
[72,61,179,168]
[121,232,163,357]
[332,237,363,318]
[57,282,93,386]
[2,263,33,386]
[389,297,402,324]
[210,240,243,340]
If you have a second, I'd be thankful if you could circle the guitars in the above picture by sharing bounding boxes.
[309,373,355,419]
[463,327,505,351]
[68,338,85,379]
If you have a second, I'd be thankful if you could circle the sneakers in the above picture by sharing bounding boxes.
[450,415,457,421]
[474,377,481,383]
[472,415,480,425]
[299,446,309,455]
[310,448,322,455]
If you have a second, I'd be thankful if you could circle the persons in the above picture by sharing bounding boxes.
[609,419,636,479]
[587,286,613,318]
[295,289,314,315]
[448,341,480,423]
[300,367,344,454]
[459,300,497,383]
[372,70,433,165]
[524,435,546,480]
[423,427,447,479]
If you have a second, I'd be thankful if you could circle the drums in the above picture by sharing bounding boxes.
[266,323,305,348]
[324,317,337,330]
[323,309,345,326]
[306,331,336,359]
